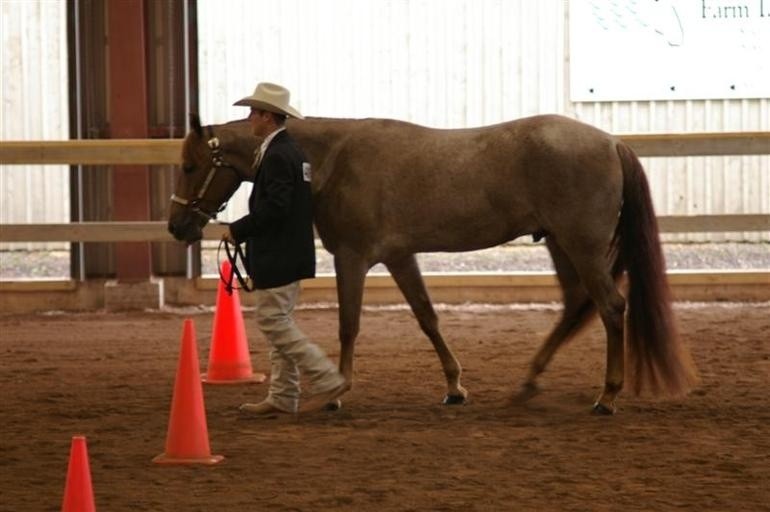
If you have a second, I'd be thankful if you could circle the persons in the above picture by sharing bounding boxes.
[222,82,351,415]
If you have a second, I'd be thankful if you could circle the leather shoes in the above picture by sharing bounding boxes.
[239,399,294,416]
[298,385,347,416]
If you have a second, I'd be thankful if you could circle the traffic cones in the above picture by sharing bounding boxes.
[196,259,268,391]
[145,312,226,471]
[57,431,96,510]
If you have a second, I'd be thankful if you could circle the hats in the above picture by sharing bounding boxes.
[233,83,306,121]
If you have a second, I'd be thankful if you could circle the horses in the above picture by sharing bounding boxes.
[167,111,700,416]
